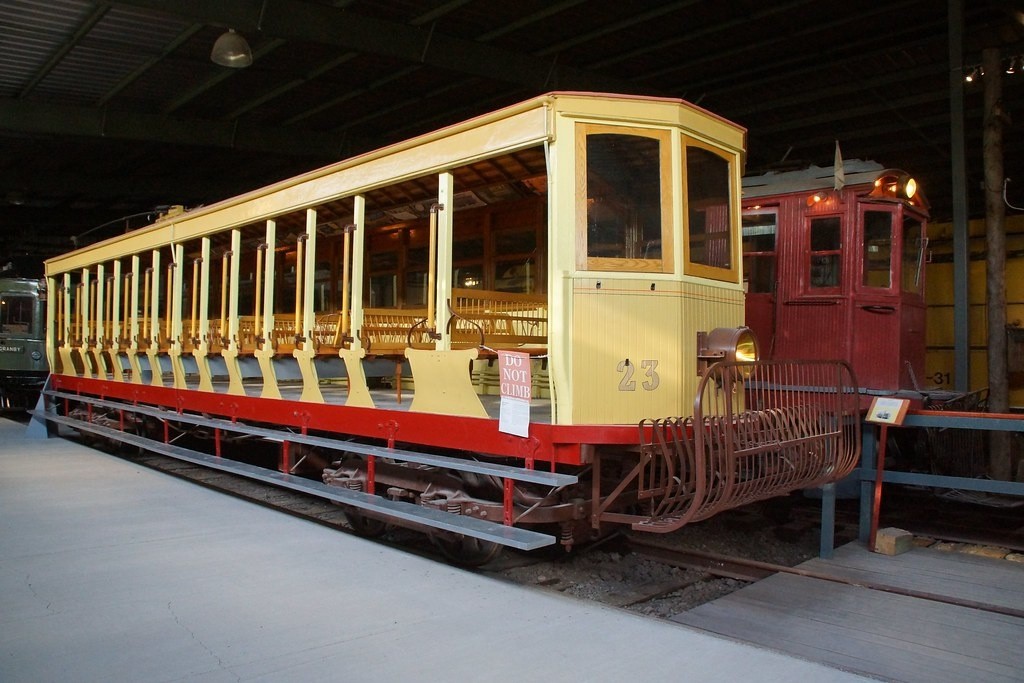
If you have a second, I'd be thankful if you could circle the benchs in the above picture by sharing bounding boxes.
[57,288,548,404]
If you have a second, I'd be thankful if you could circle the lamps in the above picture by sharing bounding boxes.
[210,28,252,68]
[966,65,977,82]
[1006,60,1015,74]
[980,66,984,75]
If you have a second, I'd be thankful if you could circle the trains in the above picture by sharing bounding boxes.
[670,136,936,415]
[43,87,865,560]
[0,277,49,412]
